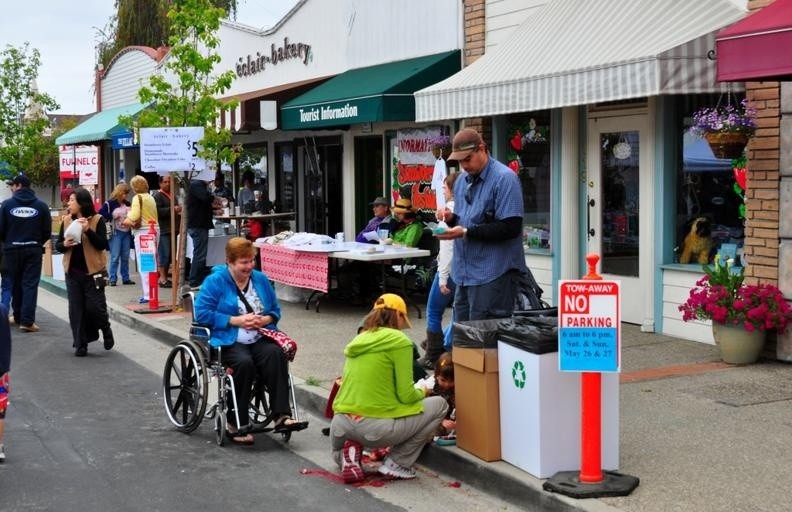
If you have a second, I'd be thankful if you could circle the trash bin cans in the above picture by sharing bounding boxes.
[43,235,66,282]
[451,316,619,480]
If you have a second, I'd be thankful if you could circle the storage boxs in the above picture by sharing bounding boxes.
[451,345,519,463]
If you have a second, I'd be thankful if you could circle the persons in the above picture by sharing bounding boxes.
[418,127,525,442]
[0,176,52,332]
[335,197,422,301]
[55,187,115,356]
[99,173,183,305]
[0,259,12,461]
[192,237,308,443]
[184,166,272,291]
[331,294,449,484]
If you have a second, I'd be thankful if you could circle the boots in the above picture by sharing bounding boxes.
[415,328,445,369]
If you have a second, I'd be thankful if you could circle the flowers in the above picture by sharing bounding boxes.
[693,97,757,139]
[676,261,792,331]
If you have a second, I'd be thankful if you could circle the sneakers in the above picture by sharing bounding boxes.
[339,438,367,484]
[139,297,150,304]
[377,455,417,480]
[8,315,40,332]
[74,343,88,356]
[100,326,114,350]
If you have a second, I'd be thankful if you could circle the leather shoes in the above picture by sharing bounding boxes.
[123,279,136,285]
[158,279,172,288]
[110,281,116,286]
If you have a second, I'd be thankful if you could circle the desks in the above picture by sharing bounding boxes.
[212,208,298,248]
[252,231,432,321]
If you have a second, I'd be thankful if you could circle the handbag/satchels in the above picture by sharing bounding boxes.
[103,200,113,240]
[119,194,142,230]
[324,375,343,419]
[255,324,299,363]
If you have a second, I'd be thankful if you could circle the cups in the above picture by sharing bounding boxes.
[234,206,241,217]
[367,247,376,254]
[221,207,230,217]
[332,232,345,250]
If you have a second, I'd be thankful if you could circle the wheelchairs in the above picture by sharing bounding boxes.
[161,290,310,448]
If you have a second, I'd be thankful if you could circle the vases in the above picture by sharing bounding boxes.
[702,130,751,159]
[712,316,770,363]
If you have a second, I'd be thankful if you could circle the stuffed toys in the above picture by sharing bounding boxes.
[679,217,718,265]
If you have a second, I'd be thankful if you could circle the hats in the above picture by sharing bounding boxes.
[388,199,414,214]
[5,175,31,188]
[368,196,390,207]
[373,293,414,330]
[445,127,483,162]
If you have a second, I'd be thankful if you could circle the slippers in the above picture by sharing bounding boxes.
[224,427,256,446]
[273,416,310,433]
